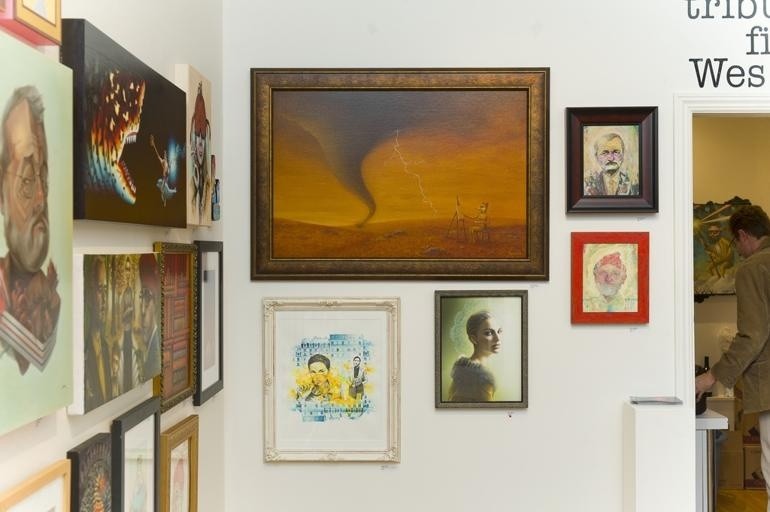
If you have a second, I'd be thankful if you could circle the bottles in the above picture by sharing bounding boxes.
[701,357,713,398]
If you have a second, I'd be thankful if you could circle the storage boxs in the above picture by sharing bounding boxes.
[700,375,767,490]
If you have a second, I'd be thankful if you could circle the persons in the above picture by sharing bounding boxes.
[296,354,343,404]
[134,253,164,382]
[695,205,770,512]
[581,251,638,314]
[189,80,212,226]
[584,132,637,197]
[447,311,503,403]
[0,86,62,374]
[348,357,368,399]
[84,256,113,408]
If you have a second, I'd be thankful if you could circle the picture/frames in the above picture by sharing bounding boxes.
[153,239,223,417]
[248,67,660,463]
[108,397,200,512]
[1,457,72,512]
